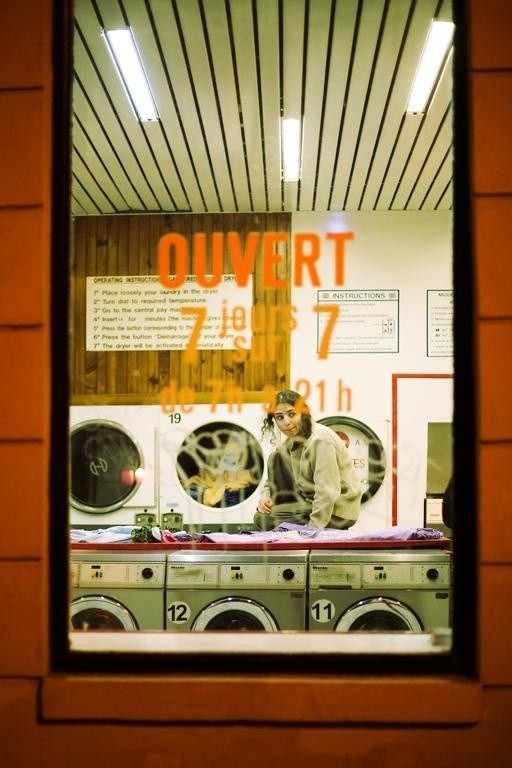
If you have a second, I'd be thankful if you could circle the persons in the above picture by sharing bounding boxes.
[253,389,362,529]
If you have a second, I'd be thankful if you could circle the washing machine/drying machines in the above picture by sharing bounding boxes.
[74,543,451,633]
[67,406,431,540]
